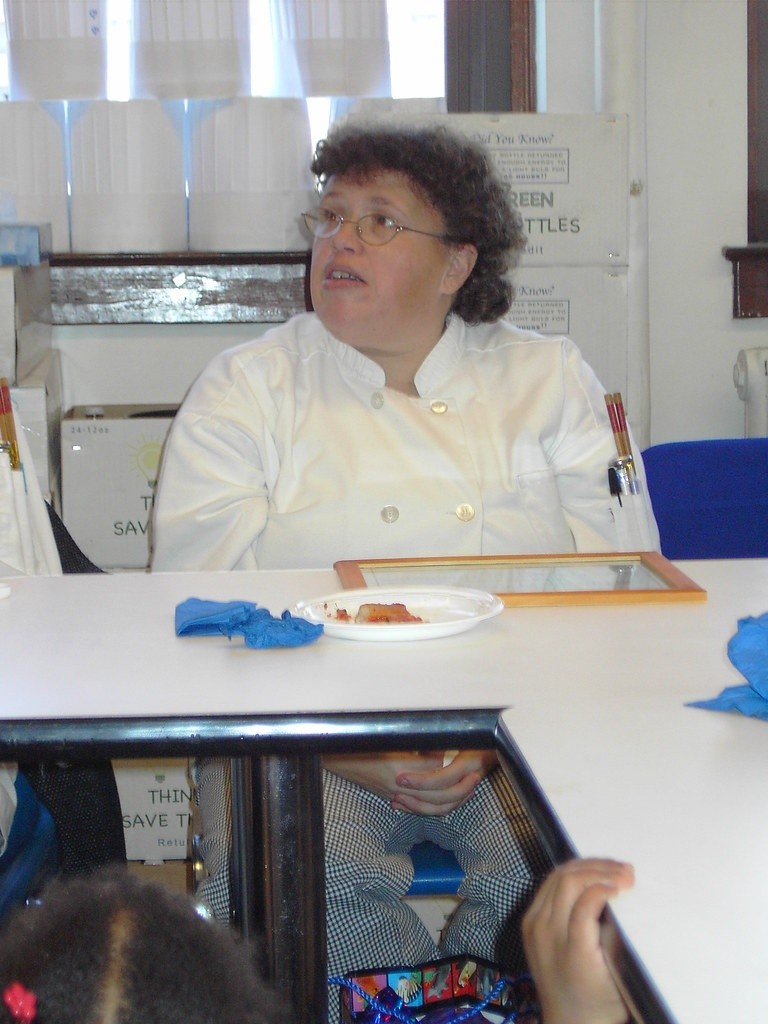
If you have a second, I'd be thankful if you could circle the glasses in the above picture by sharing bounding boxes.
[301,206,451,247]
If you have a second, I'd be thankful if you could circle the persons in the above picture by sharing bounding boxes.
[0,860,637,1024]
[149,129,659,1024]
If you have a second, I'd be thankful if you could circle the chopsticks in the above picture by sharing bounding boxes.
[605,393,632,458]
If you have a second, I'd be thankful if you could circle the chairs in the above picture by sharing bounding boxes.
[634,436,768,560]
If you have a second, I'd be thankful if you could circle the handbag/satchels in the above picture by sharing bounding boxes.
[327,953,542,1024]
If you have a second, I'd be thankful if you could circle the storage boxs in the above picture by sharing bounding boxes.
[411,110,632,451]
[61,400,182,572]
[106,755,199,870]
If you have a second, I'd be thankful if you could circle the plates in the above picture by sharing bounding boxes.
[292,586,504,640]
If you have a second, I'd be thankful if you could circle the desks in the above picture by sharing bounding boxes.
[0,558,767,1024]
